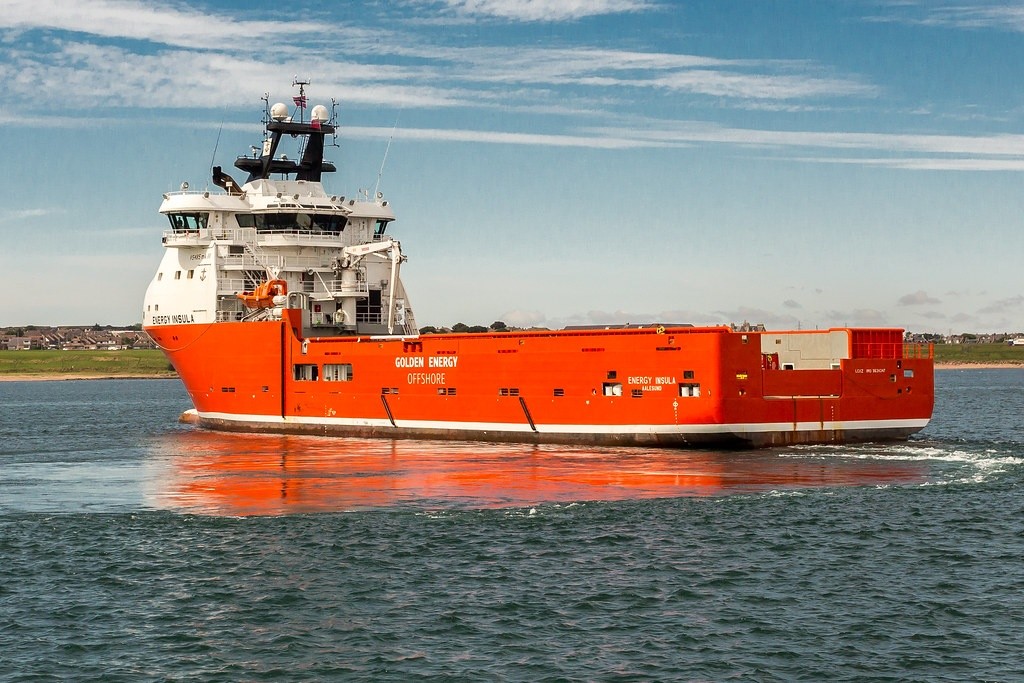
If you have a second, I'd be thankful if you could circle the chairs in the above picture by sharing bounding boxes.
[270,225,292,233]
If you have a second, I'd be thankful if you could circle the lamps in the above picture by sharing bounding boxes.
[331,195,337,201]
[377,192,383,198]
[203,192,210,198]
[181,181,189,190]
[162,193,168,200]
[337,259,350,268]
[294,193,299,199]
[277,193,282,198]
[340,196,345,202]
[306,267,314,275]
[349,200,354,205]
[382,201,388,206]
[239,193,247,199]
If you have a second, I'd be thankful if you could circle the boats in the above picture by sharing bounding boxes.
[139,75,936,449]
[237,280,287,308]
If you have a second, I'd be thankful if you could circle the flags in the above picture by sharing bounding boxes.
[293,96,306,109]
